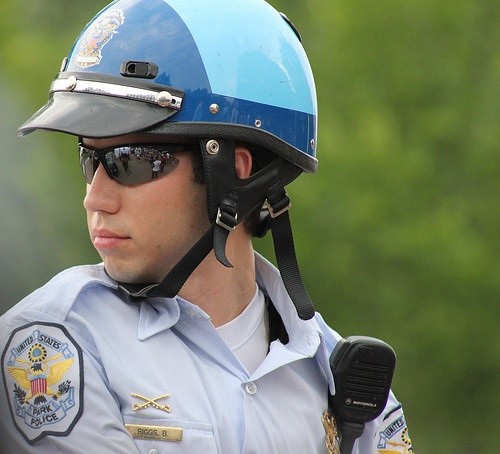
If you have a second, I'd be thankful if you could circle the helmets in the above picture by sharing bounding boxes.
[63,1,318,175]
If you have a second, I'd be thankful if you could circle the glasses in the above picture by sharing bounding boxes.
[77,139,201,185]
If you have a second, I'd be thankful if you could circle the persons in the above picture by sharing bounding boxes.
[106,147,176,181]
[0,0,415,454]
